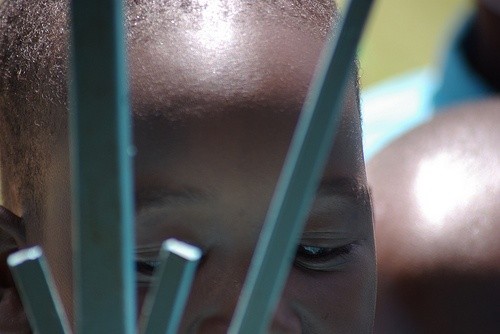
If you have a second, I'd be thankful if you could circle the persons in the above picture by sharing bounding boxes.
[0,0,379,334]
